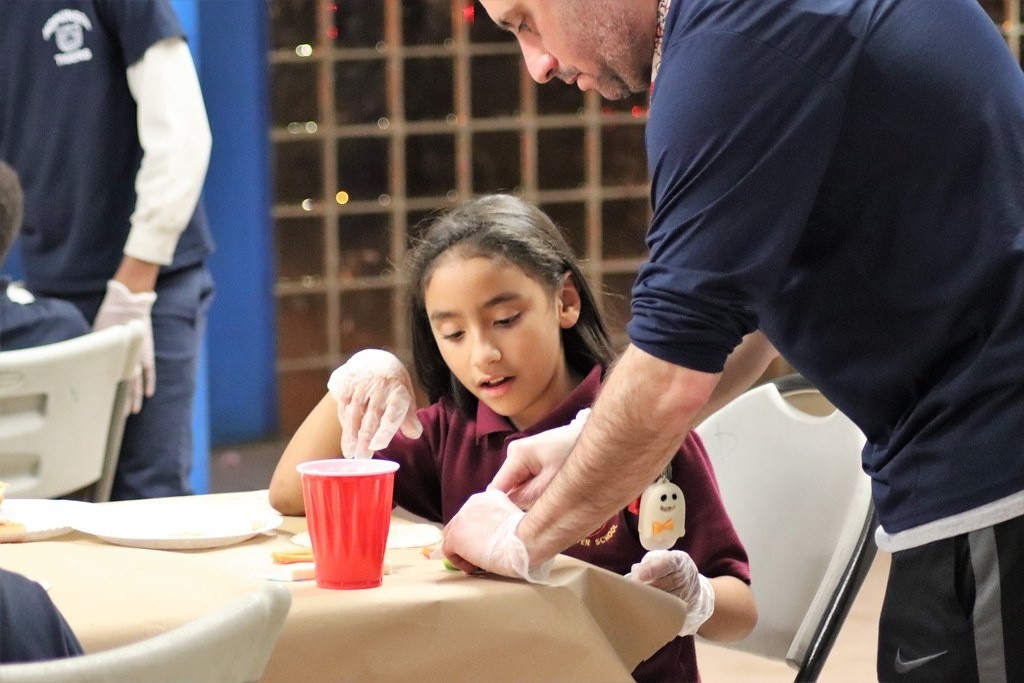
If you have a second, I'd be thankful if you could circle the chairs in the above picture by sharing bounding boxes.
[0,320,143,503]
[691,373,882,683]
[0,586,294,683]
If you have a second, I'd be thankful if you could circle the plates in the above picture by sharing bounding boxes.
[71,508,284,551]
[0,499,95,544]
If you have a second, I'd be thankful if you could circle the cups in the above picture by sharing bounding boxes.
[296,461,401,590]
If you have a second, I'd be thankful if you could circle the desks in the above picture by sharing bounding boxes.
[0,487,688,683]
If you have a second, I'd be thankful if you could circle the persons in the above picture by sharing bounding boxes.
[0,0,213,504]
[269,192,758,683]
[440,0,1024,683]
[0,159,95,352]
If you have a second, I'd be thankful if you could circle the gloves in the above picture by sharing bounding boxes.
[91,280,157,415]
